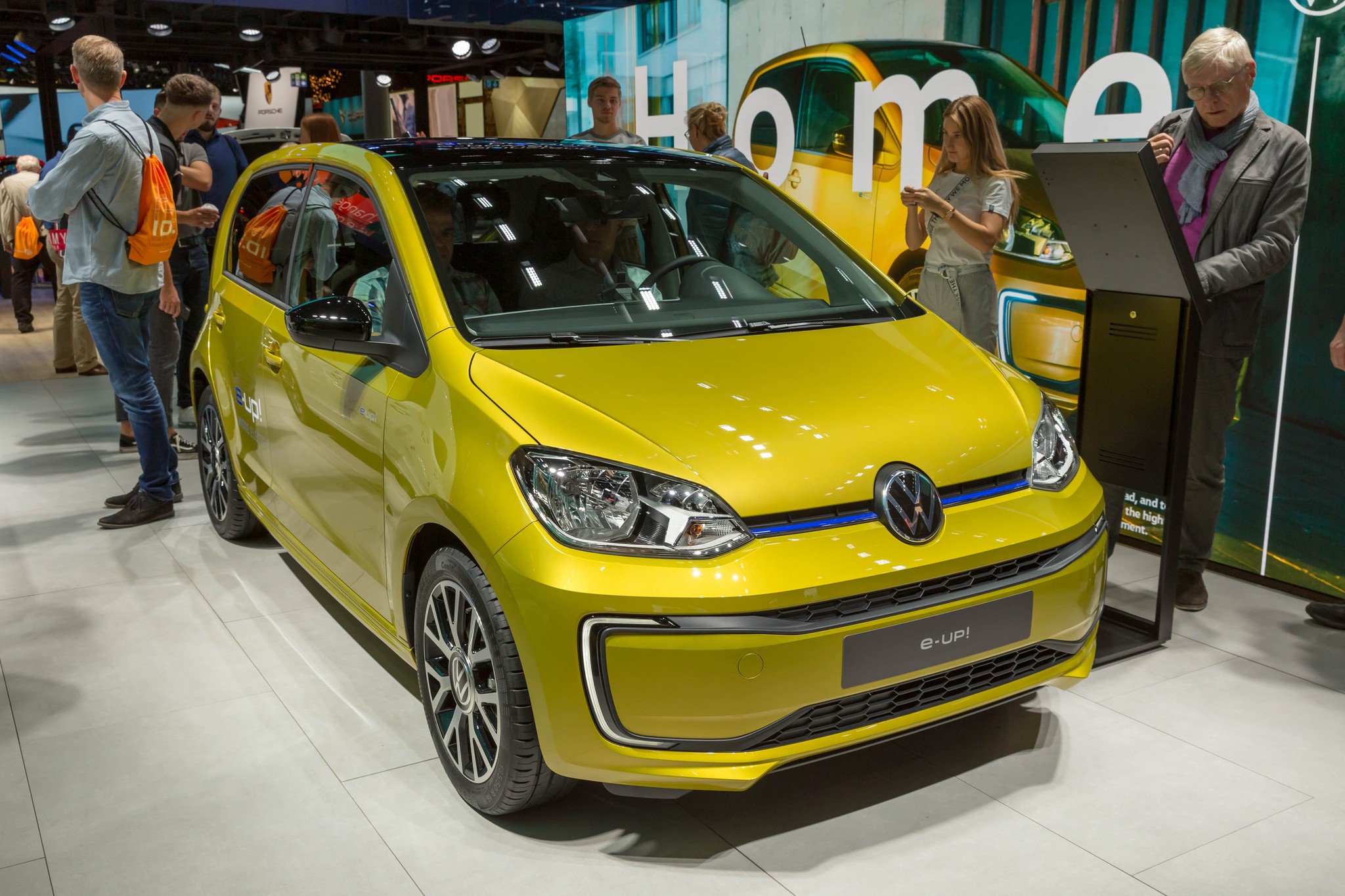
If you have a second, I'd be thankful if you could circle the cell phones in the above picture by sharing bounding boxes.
[179,304,191,321]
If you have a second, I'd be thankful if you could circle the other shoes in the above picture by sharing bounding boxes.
[1305,601,1345,630]
[177,405,196,428]
[78,363,109,376]
[55,364,77,373]
[98,493,176,527]
[103,480,184,507]
[18,323,35,333]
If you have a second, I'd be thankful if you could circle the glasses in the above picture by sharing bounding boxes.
[1186,64,1249,101]
[684,124,700,141]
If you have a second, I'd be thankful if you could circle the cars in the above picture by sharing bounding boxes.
[733,37,1248,427]
[189,132,1109,819]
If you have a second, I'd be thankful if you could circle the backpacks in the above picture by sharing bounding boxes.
[75,113,179,267]
[749,160,800,265]
[237,185,333,284]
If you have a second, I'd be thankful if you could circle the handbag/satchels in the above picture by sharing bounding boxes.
[3,180,44,260]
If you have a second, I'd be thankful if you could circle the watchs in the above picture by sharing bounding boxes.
[942,207,956,222]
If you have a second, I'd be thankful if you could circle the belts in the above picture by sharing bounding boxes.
[173,235,206,250]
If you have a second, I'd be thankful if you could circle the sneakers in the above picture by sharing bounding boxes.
[168,432,198,460]
[118,434,139,452]
[1174,568,1208,611]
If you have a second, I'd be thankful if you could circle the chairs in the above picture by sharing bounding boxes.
[355,221,394,276]
[807,68,858,147]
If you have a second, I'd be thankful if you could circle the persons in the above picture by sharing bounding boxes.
[685,103,779,289]
[0,154,57,335]
[25,34,182,530]
[347,186,502,344]
[38,150,109,376]
[1306,312,1345,631]
[564,76,647,147]
[1103,24,1312,612]
[300,113,342,145]
[900,96,1032,355]
[235,174,360,309]
[115,73,248,455]
[520,179,663,310]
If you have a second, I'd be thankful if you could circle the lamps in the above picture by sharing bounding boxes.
[490,62,510,79]
[517,57,535,75]
[237,16,263,42]
[449,34,500,60]
[262,67,280,82]
[45,0,77,31]
[0,29,41,64]
[466,65,485,82]
[544,55,564,71]
[146,13,173,37]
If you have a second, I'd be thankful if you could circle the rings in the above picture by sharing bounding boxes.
[1159,148,1162,154]
